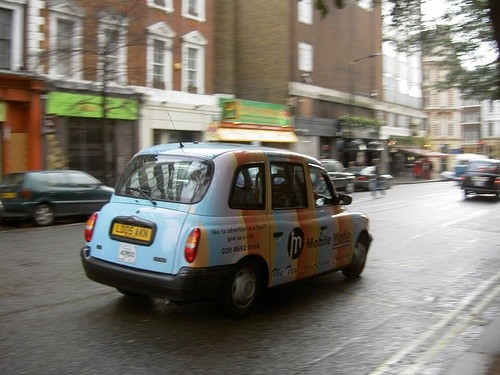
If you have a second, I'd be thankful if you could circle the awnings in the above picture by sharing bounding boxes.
[400,148,452,159]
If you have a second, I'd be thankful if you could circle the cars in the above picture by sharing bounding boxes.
[318,158,354,192]
[1,168,115,226]
[82,110,371,317]
[343,165,393,190]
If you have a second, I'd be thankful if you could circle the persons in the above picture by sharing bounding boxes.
[370,158,386,197]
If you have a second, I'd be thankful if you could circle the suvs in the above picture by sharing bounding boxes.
[454,155,487,178]
[462,159,500,199]
